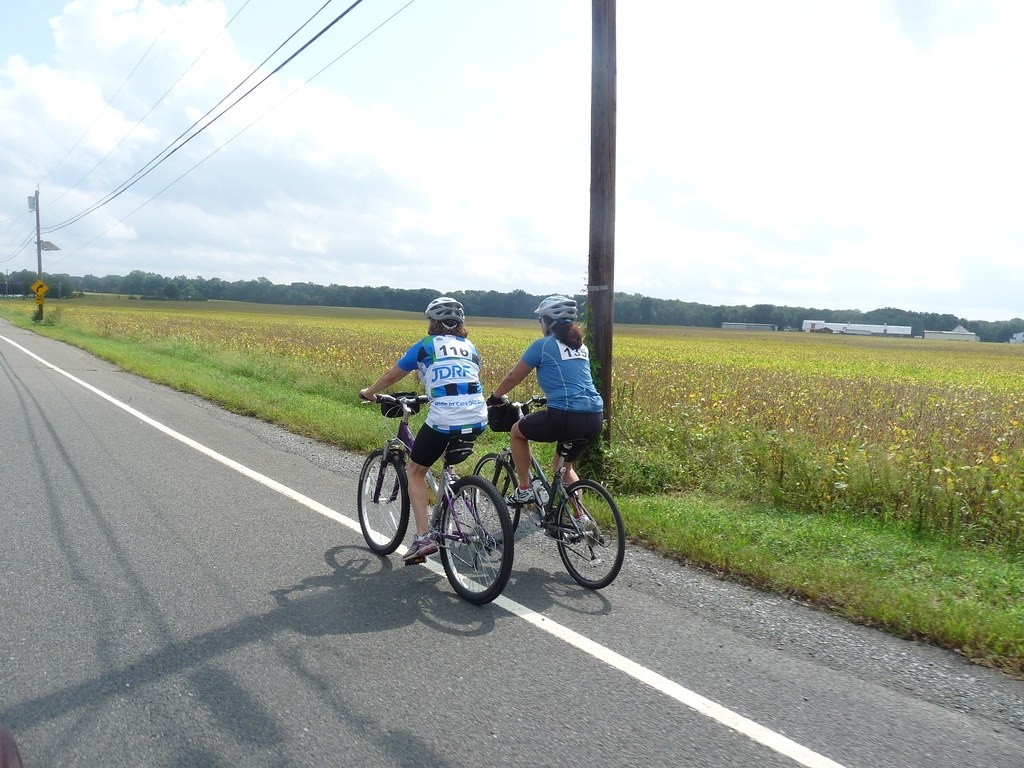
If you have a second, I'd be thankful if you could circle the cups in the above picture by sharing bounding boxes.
[531,475,549,509]
[424,475,438,507]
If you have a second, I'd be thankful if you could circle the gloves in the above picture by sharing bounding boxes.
[486,394,508,410]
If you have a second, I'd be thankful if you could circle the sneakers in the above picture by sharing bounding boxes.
[402,532,438,561]
[563,514,590,529]
[504,487,534,505]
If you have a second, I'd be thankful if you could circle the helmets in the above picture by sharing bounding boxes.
[425,297,465,323]
[534,296,578,320]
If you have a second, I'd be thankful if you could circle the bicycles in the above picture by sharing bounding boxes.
[357,392,514,604]
[471,395,625,590]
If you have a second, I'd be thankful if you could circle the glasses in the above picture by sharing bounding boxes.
[539,317,544,323]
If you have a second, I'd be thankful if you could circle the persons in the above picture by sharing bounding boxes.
[359,297,488,561]
[486,296,603,538]
